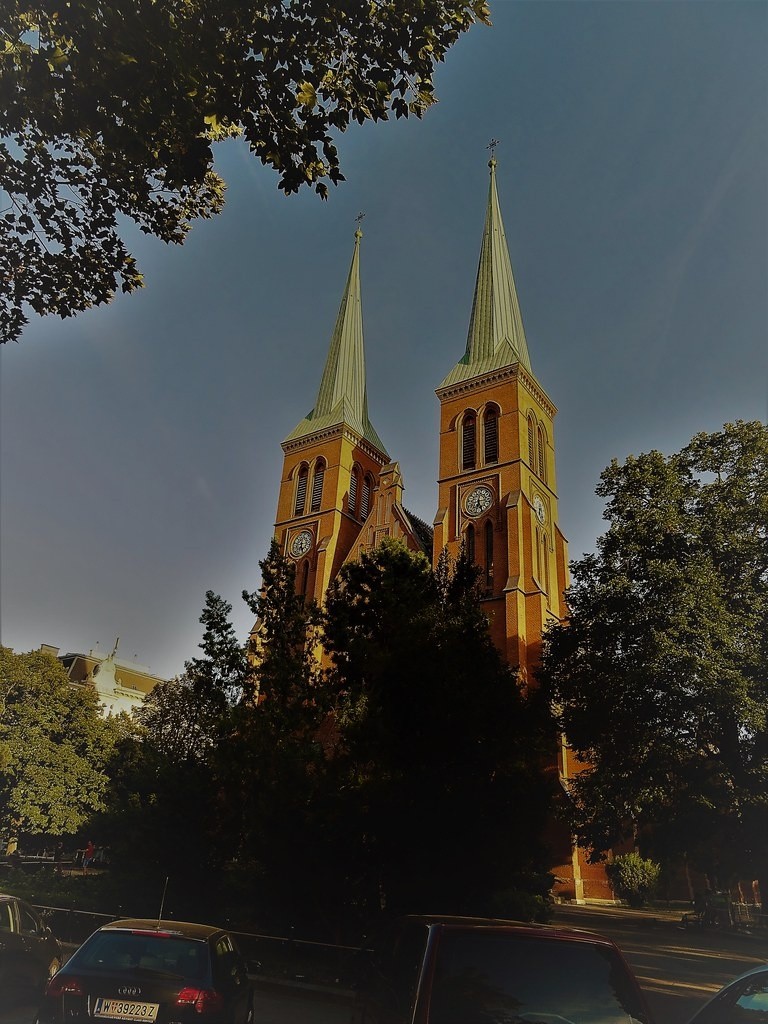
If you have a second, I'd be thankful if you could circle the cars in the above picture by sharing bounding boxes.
[685,962,768,1023]
[35,917,255,1023]
[350,913,658,1024]
[0,891,63,993]
[551,874,575,904]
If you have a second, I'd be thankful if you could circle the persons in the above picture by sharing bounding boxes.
[77,840,94,875]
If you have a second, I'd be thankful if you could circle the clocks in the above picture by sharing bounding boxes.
[287,527,314,560]
[532,493,547,526]
[465,486,493,517]
